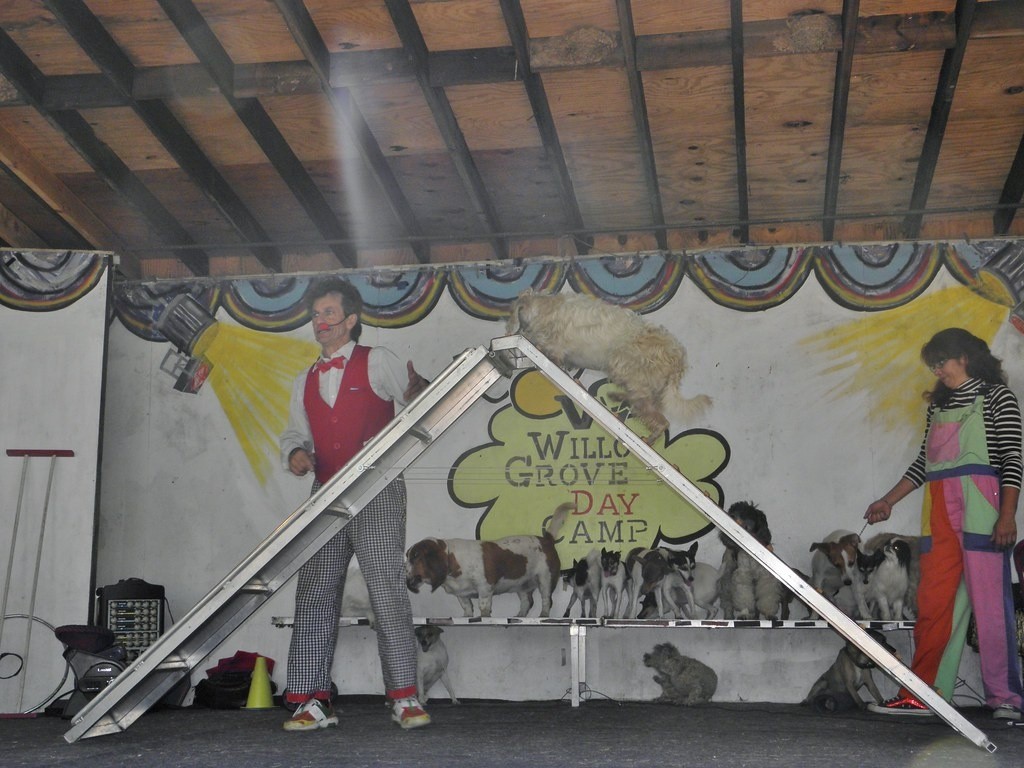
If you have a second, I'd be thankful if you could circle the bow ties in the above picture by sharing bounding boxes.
[316,355,346,373]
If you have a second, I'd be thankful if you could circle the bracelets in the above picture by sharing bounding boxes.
[880,498,894,507]
[289,447,301,458]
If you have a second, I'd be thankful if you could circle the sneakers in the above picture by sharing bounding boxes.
[283,697,339,731]
[391,695,431,729]
[868,694,934,715]
[993,702,1021,719]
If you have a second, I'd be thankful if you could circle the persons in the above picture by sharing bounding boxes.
[863,327,1024,721]
[278,277,431,732]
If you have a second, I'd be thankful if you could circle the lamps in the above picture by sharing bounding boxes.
[977,242,1024,307]
[154,292,220,396]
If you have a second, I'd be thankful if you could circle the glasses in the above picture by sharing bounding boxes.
[930,356,951,373]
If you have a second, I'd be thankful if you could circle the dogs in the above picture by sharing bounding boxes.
[506,289,716,446]
[384,624,462,708]
[643,641,718,706]
[563,500,912,622]
[799,629,897,713]
[405,530,565,618]
[339,568,378,630]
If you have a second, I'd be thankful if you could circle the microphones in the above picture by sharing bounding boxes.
[319,317,347,330]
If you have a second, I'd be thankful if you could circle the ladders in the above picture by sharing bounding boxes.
[59,328,1003,756]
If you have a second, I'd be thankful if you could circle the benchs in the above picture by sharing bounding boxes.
[270,617,917,708]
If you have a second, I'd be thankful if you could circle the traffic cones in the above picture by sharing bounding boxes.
[237,656,282,709]
